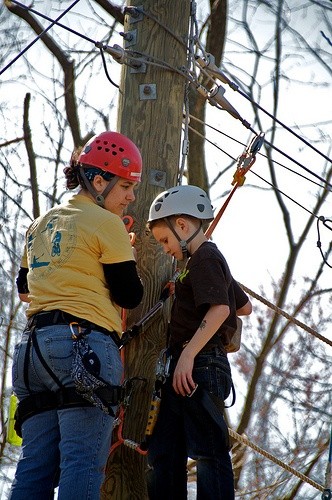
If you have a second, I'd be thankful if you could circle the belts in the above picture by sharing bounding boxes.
[23,310,121,346]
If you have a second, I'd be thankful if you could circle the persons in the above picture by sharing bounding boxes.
[143,186,254,500]
[7,131,143,500]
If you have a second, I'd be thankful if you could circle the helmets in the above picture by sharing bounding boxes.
[147,185,215,224]
[76,131,143,182]
